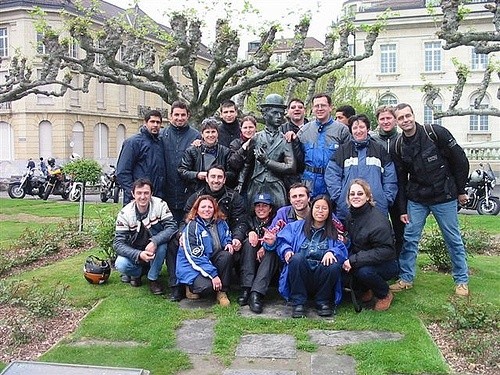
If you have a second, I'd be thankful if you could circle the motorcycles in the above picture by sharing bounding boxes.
[456,156,500,216]
[42,158,70,199]
[64,154,83,202]
[7,166,46,199]
[98,163,123,205]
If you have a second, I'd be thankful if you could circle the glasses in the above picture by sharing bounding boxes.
[349,191,368,197]
[289,105,305,111]
[314,104,330,110]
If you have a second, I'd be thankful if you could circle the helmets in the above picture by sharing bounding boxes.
[82,261,113,286]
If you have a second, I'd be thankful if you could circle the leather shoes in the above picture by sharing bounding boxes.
[236,289,248,307]
[129,278,140,287]
[292,304,306,319]
[316,302,332,317]
[149,280,164,294]
[249,292,263,314]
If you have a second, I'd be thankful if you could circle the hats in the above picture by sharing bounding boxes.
[253,192,274,206]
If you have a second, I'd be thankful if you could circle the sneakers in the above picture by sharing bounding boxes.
[374,291,393,313]
[217,289,231,307]
[185,286,199,301]
[388,277,413,292]
[168,287,182,302]
[454,283,469,296]
[361,289,374,303]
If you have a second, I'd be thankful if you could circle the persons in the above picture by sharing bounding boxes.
[235,93,298,222]
[257,183,351,307]
[175,195,236,308]
[25,155,48,174]
[334,105,361,134]
[158,100,203,216]
[110,178,178,294]
[325,112,398,243]
[392,103,471,301]
[284,92,352,214]
[275,194,351,318]
[166,164,248,303]
[278,97,314,135]
[342,179,400,310]
[177,118,234,215]
[233,193,284,314]
[191,101,240,149]
[368,104,408,275]
[227,116,257,196]
[115,110,168,283]
